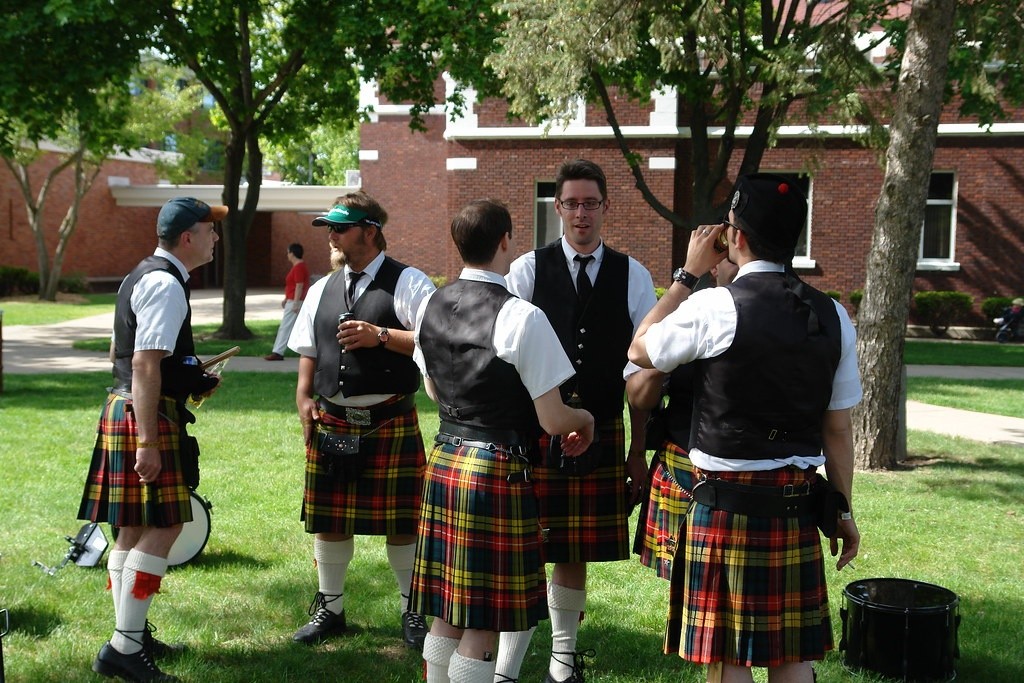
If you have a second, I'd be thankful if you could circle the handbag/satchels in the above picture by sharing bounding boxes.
[813,473,849,537]
[321,434,364,479]
[181,437,200,491]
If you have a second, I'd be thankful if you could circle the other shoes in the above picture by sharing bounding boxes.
[265,352,284,361]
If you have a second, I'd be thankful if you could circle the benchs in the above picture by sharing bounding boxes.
[85,276,124,291]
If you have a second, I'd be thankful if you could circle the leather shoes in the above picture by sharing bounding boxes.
[92,642,181,683]
[402,610,429,649]
[140,622,190,664]
[293,606,346,644]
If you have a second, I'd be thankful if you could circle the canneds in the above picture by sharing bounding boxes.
[338,313,357,346]
[180,356,198,365]
[624,480,641,517]
[713,227,729,254]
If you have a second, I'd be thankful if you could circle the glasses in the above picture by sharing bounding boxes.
[724,217,747,236]
[559,198,604,210]
[327,223,366,233]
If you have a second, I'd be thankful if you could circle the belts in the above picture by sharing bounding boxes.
[320,393,417,427]
[438,421,540,448]
[112,378,187,405]
[692,480,822,518]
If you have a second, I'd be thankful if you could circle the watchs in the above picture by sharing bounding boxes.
[673,268,699,290]
[377,327,389,347]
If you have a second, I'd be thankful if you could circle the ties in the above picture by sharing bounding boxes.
[573,256,595,302]
[345,271,367,310]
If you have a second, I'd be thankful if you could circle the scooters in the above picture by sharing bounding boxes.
[993,304,1024,343]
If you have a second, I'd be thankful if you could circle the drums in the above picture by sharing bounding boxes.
[108,486,213,570]
[835,577,962,683]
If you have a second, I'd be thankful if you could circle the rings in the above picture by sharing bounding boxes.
[703,230,709,235]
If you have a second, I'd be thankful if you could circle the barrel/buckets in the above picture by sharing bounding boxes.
[838,577,960,683]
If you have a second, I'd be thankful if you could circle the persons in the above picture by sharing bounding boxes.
[494,159,658,683]
[76,197,229,683]
[264,243,310,361]
[406,200,594,683]
[993,297,1024,326]
[624,175,863,683]
[286,191,436,647]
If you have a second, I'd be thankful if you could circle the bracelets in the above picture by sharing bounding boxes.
[838,512,854,520]
[137,442,160,448]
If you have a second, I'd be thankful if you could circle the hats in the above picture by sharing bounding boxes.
[312,204,381,229]
[732,173,808,242]
[157,197,229,239]
